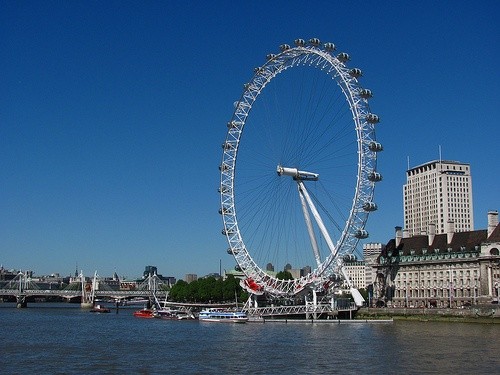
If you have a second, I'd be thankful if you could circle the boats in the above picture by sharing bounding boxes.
[199,311,248,323]
[88,297,196,323]
